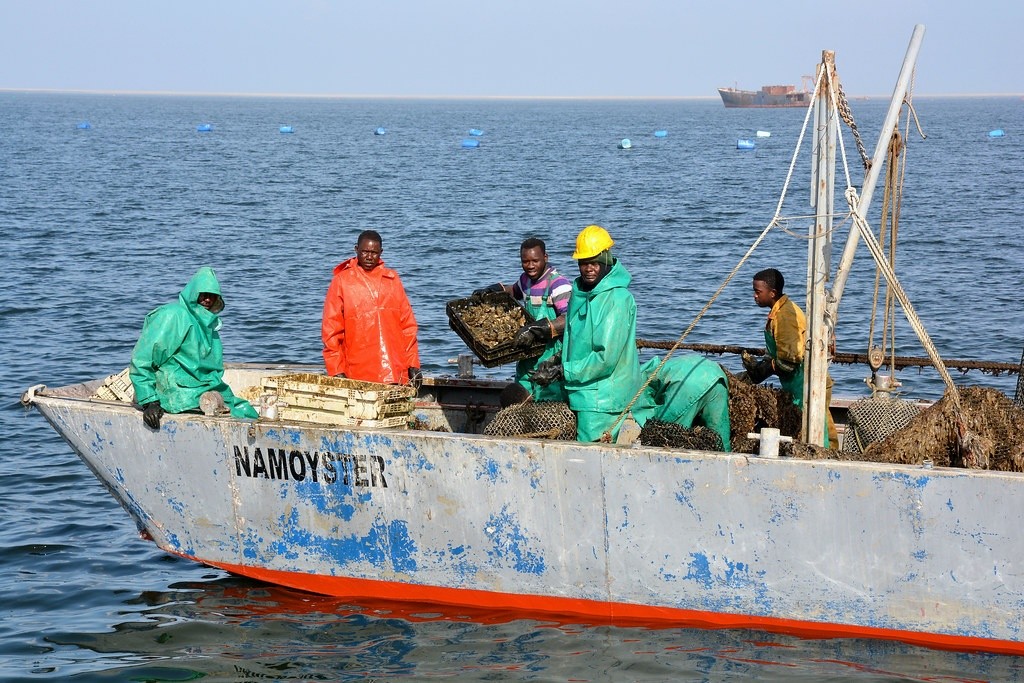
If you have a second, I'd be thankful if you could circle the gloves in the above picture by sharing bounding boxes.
[539,351,561,373]
[744,357,776,385]
[143,401,164,430]
[472,283,506,306]
[334,373,346,379]
[528,361,564,388]
[409,367,423,391]
[511,317,551,352]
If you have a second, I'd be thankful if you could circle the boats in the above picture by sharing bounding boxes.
[717,85,816,109]
[26,364,1024,657]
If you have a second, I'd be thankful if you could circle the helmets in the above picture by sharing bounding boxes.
[571,225,615,259]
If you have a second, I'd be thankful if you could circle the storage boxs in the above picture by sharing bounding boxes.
[276,373,413,431]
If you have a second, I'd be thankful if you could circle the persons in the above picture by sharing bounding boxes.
[528,225,657,443]
[741,268,840,450]
[128,266,259,429]
[471,238,573,402]
[640,354,730,452]
[322,231,423,393]
